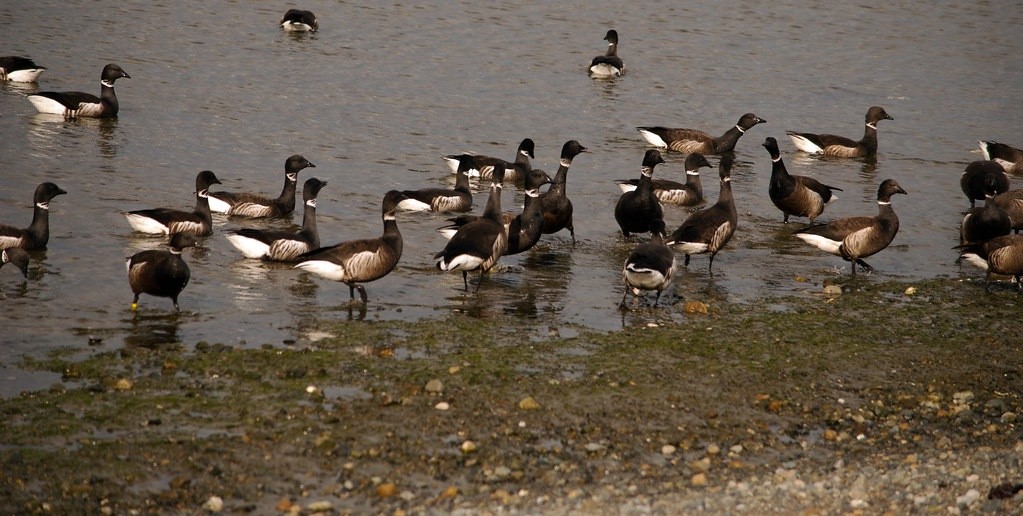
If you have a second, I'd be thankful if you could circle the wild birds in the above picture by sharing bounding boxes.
[586,29,627,79]
[279,8,319,34]
[0,55,133,116]
[615,105,907,311]
[0,181,67,278]
[953,137,1023,285]
[122,137,587,318]
